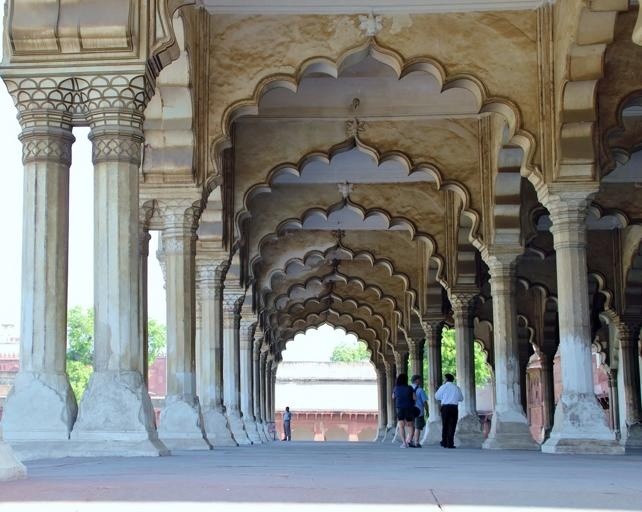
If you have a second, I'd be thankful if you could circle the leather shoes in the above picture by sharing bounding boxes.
[440,440,446,446]
[447,445,456,449]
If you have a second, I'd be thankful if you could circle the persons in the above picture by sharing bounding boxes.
[409,375,430,448]
[282,407,291,441]
[435,374,464,448]
[392,373,417,448]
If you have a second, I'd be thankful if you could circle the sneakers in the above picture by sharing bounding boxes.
[400,443,407,449]
